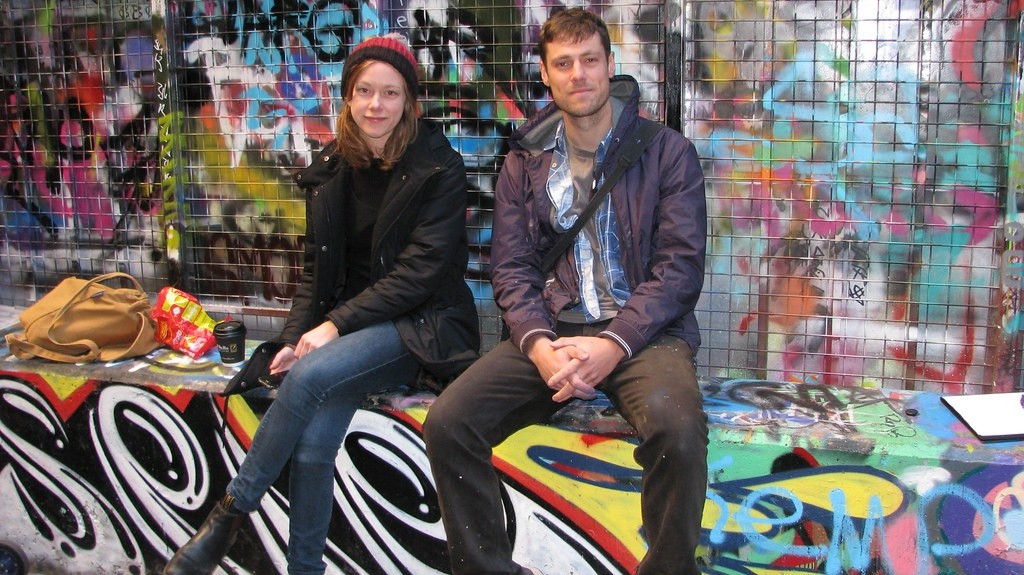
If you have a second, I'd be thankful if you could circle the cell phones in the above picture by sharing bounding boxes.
[257,369,289,390]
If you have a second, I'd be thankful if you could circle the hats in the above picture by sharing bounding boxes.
[340,32,421,94]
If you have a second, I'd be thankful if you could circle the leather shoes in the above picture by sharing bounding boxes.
[163,499,244,575]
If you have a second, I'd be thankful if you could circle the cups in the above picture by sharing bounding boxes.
[213,320,246,367]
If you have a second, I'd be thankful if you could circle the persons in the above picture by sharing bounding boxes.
[166,34,482,575]
[424,9,709,575]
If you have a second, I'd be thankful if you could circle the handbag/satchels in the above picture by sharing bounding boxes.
[6,271,160,364]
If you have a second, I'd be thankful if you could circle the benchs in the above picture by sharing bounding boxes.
[0,304,1024,575]
